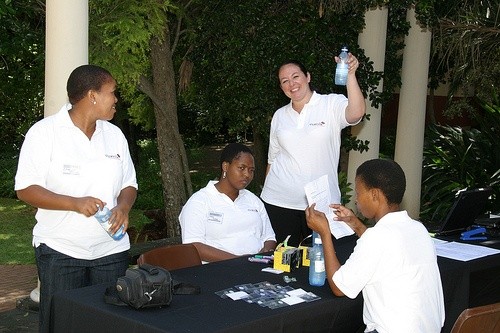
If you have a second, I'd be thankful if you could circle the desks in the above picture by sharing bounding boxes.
[44,228,500,333]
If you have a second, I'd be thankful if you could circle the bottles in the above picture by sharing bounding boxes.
[334,46,349,86]
[93,204,132,242]
[308,237,327,286]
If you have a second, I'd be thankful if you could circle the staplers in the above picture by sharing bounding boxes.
[460,228,487,240]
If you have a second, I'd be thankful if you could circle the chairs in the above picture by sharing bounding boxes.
[137,243,202,273]
[450,302,500,333]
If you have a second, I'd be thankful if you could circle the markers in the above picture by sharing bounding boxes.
[255,255,274,259]
[248,257,274,264]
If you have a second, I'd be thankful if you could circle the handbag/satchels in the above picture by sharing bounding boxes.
[102,263,202,309]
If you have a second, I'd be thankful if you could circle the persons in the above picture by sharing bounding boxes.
[14,65,138,333]
[178,144,277,265]
[305,158,445,333]
[259,52,366,247]
